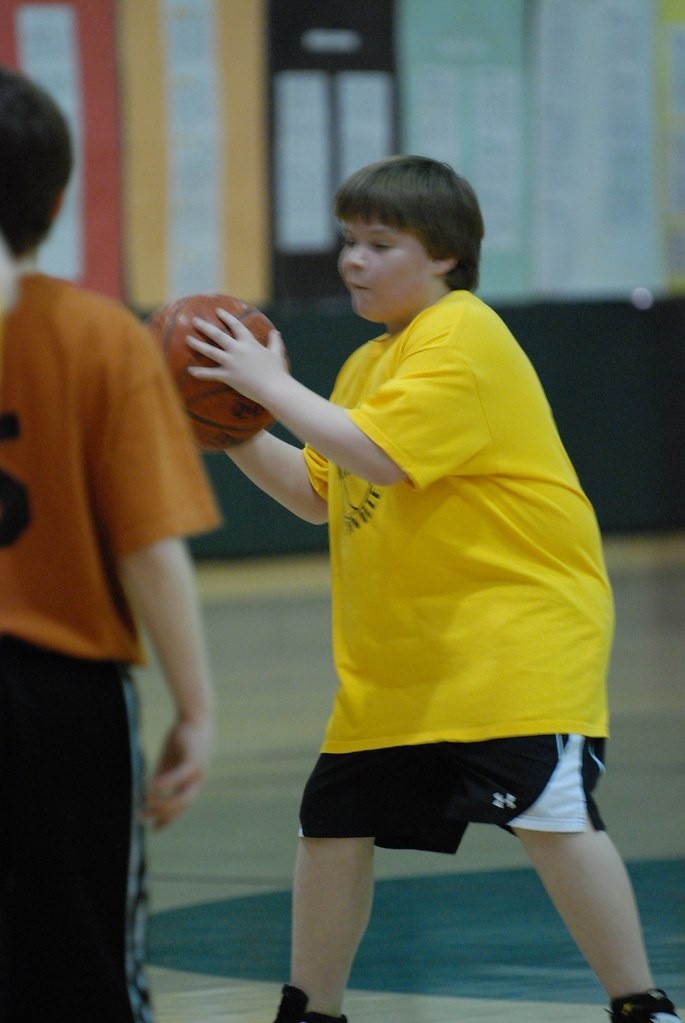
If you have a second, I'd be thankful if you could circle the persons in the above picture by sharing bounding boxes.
[0,67,224,1023]
[184,155,682,1023]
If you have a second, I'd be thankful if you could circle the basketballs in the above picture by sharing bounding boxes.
[144,291,291,456]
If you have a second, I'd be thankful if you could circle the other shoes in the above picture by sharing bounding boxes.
[272,984,348,1023]
[603,988,682,1023]
